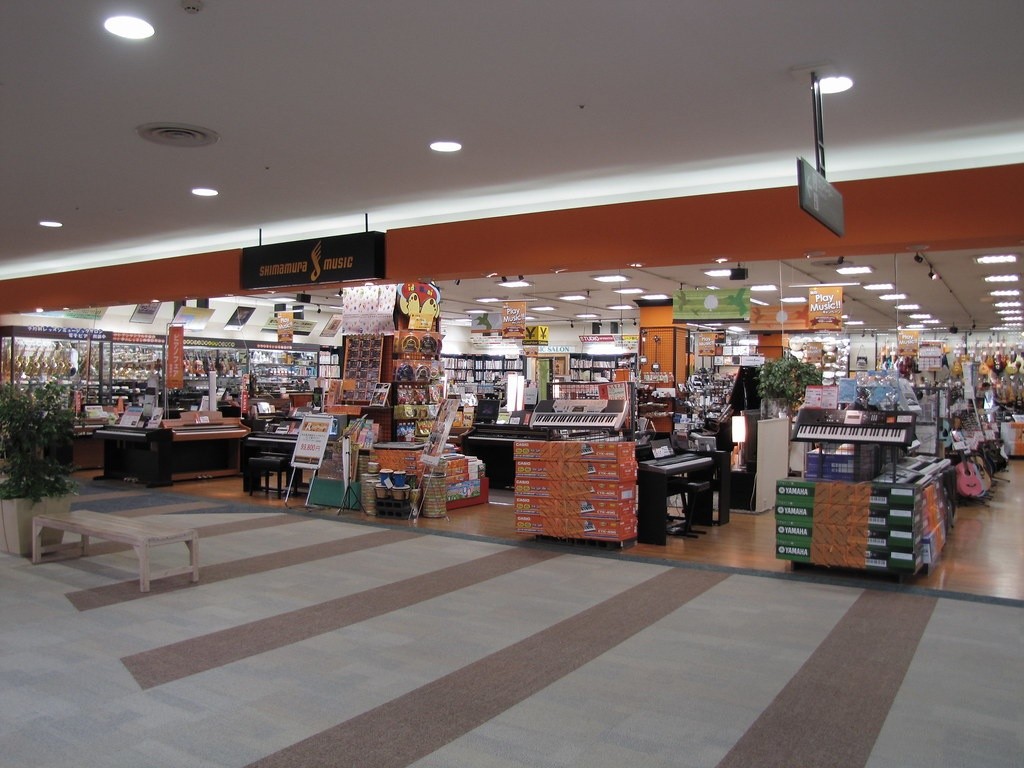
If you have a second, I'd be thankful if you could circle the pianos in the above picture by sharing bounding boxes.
[93,425,174,488]
[529,399,629,431]
[635,431,732,545]
[160,411,251,481]
[791,409,918,447]
[243,417,319,497]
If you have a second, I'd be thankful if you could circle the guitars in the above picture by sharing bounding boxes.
[952,410,1010,500]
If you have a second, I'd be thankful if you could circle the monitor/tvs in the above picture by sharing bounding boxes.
[477,400,502,423]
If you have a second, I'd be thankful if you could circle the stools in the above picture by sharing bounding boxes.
[667,480,710,538]
[248,456,298,499]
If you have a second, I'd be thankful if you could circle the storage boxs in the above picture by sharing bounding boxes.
[515,514,567,538]
[370,449,485,484]
[514,440,564,461]
[565,499,637,520]
[563,461,638,483]
[566,515,637,540]
[774,448,946,576]
[564,441,635,463]
[514,496,566,517]
[516,460,564,481]
[564,481,637,502]
[515,478,565,499]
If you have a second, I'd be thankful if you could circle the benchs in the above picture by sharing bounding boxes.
[32,510,199,592]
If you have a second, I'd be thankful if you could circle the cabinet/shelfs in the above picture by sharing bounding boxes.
[0,325,637,417]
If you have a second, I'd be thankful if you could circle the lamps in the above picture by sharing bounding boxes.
[632,319,637,327]
[732,416,746,470]
[619,319,623,327]
[571,321,574,328]
[599,320,602,327]
[729,261,748,279]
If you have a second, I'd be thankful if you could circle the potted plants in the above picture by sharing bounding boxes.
[0,381,87,558]
